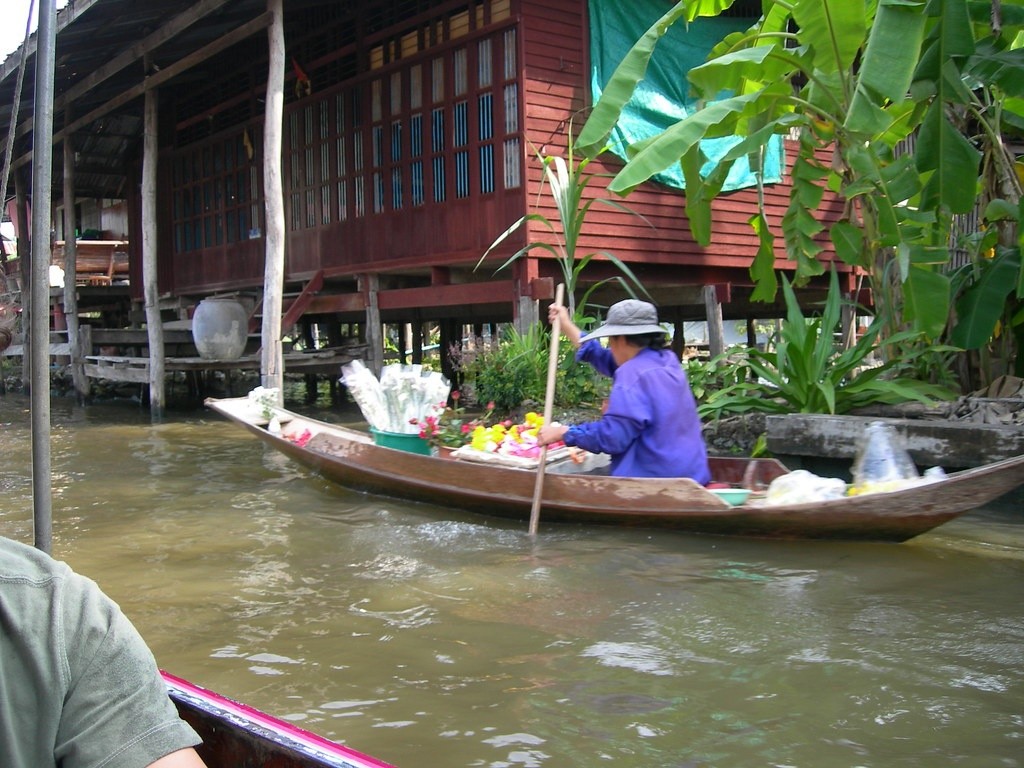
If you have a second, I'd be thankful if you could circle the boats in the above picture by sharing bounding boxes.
[203,387,1024,544]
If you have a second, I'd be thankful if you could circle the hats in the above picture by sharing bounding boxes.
[578,299,668,343]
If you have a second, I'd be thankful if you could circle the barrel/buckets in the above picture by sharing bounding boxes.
[370,428,431,457]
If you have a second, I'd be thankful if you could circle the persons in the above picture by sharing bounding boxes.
[537,299,711,487]
[0,535,208,768]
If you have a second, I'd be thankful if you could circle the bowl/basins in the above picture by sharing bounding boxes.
[708,489,752,505]
